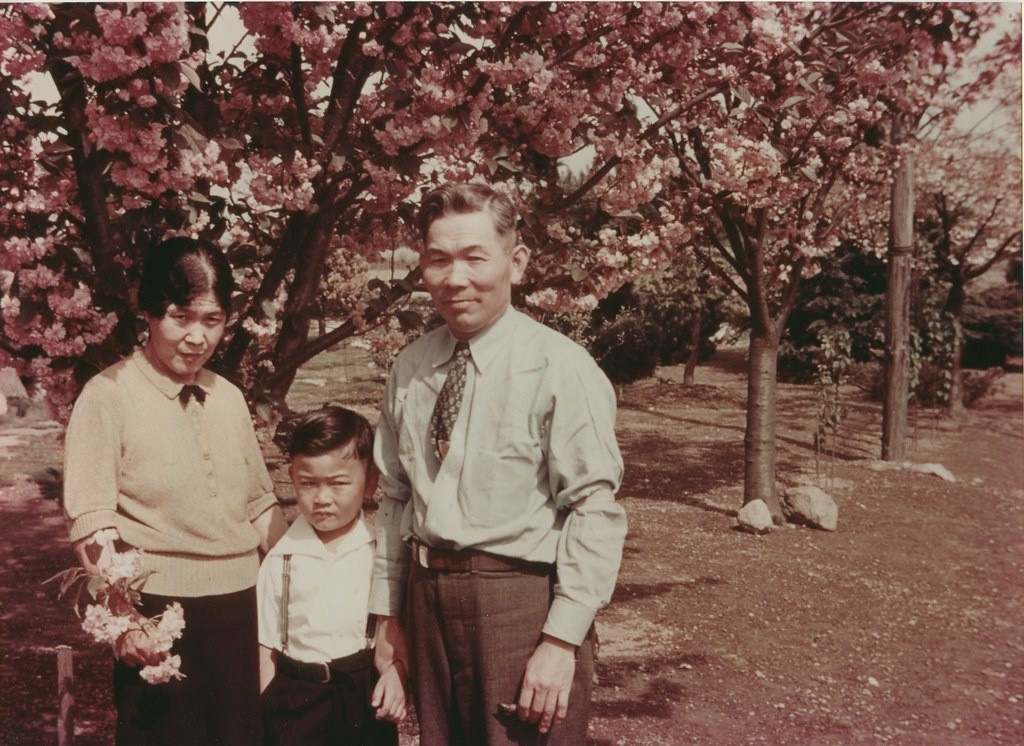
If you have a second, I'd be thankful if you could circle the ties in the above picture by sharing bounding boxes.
[429,342,472,465]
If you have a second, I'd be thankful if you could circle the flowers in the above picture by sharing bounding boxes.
[38,531,190,684]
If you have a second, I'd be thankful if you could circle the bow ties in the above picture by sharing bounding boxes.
[178,384,207,404]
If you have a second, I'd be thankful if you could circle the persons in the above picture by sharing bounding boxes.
[366,181,628,746]
[256,405,407,746]
[62,237,289,746]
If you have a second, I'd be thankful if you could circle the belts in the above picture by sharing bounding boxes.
[409,536,521,573]
[270,648,374,684]
